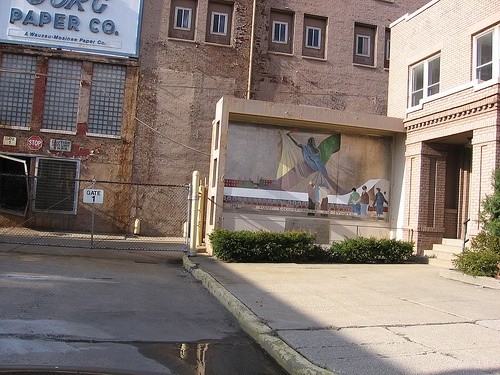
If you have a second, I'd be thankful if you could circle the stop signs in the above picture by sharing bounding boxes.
[27,136,42,150]
